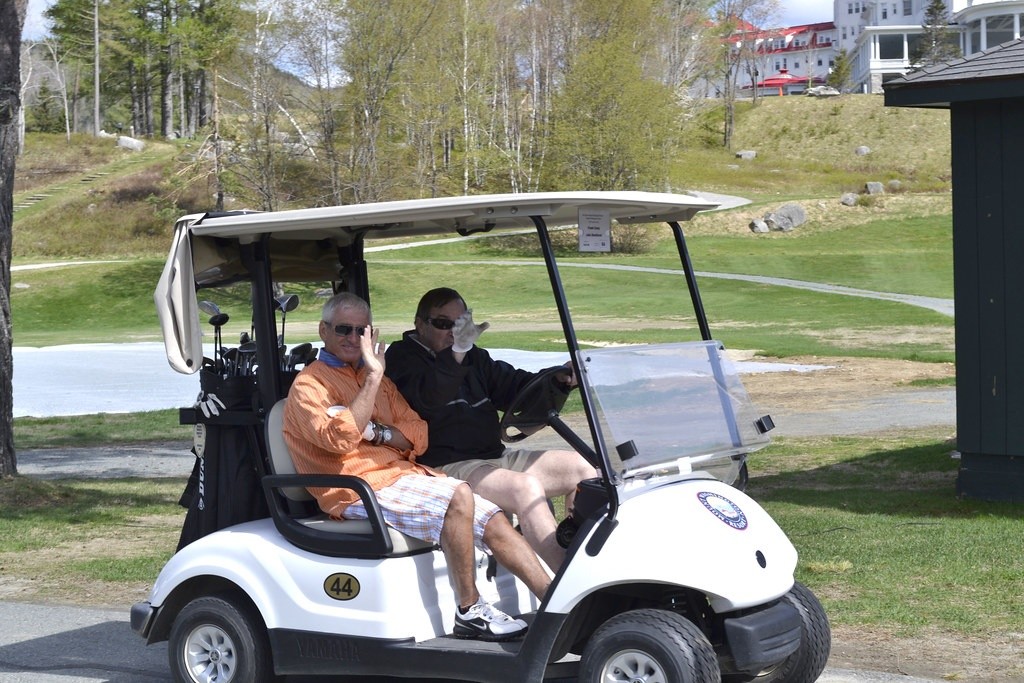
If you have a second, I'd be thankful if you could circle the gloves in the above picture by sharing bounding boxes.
[326,406,376,442]
[452,308,490,353]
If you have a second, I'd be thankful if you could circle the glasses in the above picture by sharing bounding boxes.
[418,316,455,330]
[324,321,372,338]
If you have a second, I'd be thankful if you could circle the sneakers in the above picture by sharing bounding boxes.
[453,595,529,641]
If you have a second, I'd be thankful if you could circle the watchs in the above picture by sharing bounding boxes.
[379,422,393,444]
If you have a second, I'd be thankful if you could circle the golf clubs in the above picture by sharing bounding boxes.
[200,294,318,381]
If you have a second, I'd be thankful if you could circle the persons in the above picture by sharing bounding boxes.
[282,286,602,641]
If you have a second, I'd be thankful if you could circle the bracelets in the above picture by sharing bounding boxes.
[372,421,382,446]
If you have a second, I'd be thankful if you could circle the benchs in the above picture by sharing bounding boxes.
[261,398,555,559]
